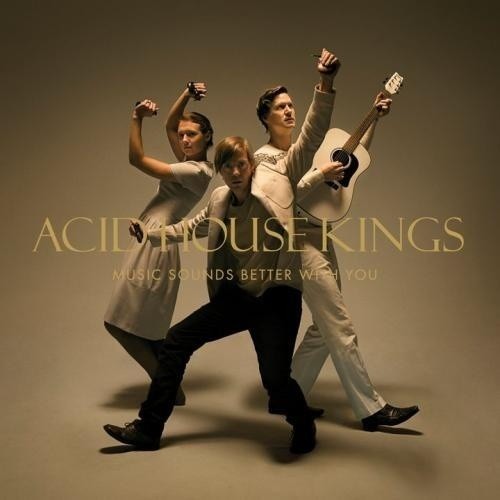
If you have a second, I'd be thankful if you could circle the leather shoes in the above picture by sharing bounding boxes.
[290,424,316,456]
[363,403,419,432]
[268,403,325,419]
[103,419,160,451]
[139,384,187,407]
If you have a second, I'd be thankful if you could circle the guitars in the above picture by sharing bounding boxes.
[294,71,404,228]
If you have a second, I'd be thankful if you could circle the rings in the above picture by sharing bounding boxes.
[335,176,341,181]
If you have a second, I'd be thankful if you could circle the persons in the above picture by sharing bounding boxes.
[253,85,422,433]
[102,80,217,406]
[101,47,342,457]
